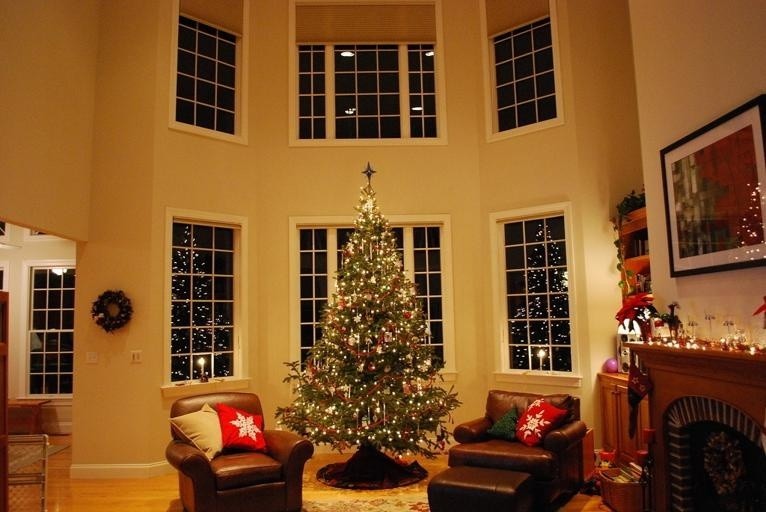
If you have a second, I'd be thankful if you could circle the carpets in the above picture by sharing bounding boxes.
[9,442,70,475]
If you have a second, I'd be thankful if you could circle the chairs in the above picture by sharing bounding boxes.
[165,392,314,512]
[446,391,587,509]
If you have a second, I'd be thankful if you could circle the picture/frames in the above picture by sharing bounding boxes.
[661,93,766,278]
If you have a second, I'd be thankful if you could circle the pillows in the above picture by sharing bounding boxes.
[486,397,569,447]
[168,403,268,465]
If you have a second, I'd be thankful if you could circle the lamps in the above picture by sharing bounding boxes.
[616,318,641,342]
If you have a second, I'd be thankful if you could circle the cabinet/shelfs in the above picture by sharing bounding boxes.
[597,372,649,466]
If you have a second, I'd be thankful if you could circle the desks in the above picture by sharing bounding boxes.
[8,398,52,436]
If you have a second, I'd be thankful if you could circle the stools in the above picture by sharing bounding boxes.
[427,465,532,511]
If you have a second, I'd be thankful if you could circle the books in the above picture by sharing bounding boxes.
[601,461,646,484]
